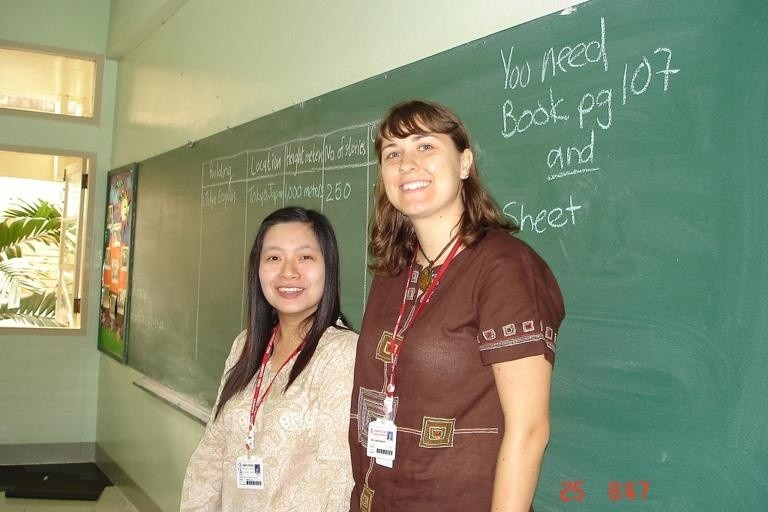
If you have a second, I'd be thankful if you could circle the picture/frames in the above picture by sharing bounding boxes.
[98,161,139,366]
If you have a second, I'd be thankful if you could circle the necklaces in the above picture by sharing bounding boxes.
[417,230,460,292]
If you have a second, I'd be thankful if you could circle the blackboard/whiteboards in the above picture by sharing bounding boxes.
[95,0,768,511]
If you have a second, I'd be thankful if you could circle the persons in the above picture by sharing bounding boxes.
[179,206,359,512]
[348,97,565,512]
